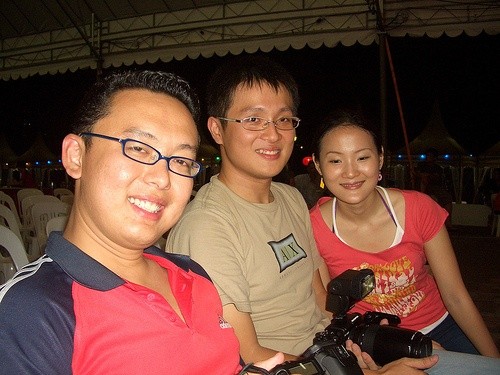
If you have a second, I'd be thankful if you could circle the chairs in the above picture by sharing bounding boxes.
[0,188,75,285]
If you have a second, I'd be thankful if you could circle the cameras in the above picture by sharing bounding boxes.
[313,269,433,371]
[269,338,364,375]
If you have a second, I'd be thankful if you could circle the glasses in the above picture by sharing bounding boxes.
[79,132,201,179]
[215,115,300,131]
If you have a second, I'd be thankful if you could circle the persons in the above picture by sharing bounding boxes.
[165,55,500,375]
[0,71,285,375]
